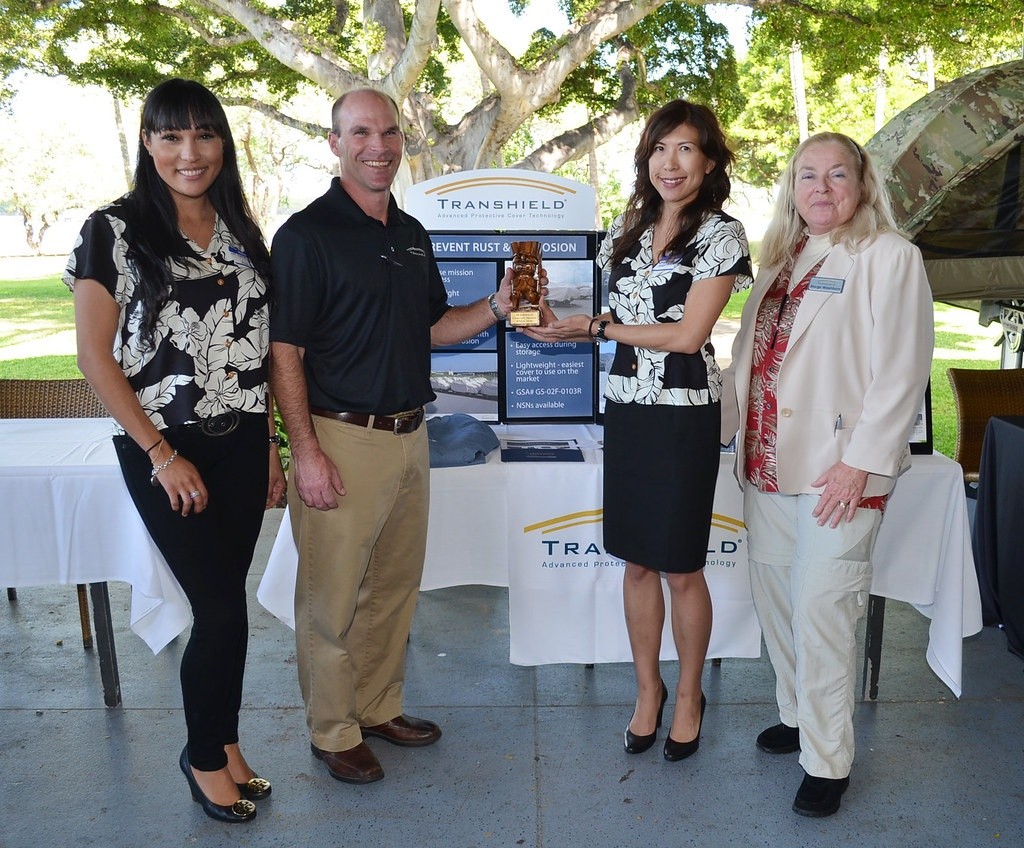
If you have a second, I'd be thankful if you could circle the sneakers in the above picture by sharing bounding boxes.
[755,723,799,755]
[791,774,849,818]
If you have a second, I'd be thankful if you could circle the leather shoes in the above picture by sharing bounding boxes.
[359,713,442,747]
[311,742,385,784]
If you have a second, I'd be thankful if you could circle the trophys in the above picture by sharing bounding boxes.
[509,240,543,326]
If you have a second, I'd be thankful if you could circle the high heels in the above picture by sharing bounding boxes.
[234,768,272,801]
[624,678,668,754]
[179,745,257,823]
[663,689,706,762]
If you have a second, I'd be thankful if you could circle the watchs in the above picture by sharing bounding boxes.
[269,432,280,445]
[593,320,610,343]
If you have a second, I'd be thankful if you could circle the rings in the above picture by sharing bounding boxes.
[190,491,200,498]
[838,501,847,509]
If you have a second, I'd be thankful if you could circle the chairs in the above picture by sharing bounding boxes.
[946,367,1024,536]
[0,378,114,650]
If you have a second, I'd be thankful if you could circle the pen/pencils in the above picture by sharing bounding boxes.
[381,255,404,267]
[834,413,842,438]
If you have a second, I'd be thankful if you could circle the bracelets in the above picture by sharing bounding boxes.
[146,436,166,463]
[151,449,178,487]
[589,319,598,337]
[488,294,508,320]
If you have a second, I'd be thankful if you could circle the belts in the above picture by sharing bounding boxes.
[160,411,267,436]
[308,404,424,437]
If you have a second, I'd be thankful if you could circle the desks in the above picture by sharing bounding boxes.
[1,415,192,708]
[255,422,983,704]
[971,413,1024,660]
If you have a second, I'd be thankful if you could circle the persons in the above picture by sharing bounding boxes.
[62,78,288,823]
[272,89,513,784]
[519,98,754,761]
[722,132,935,817]
